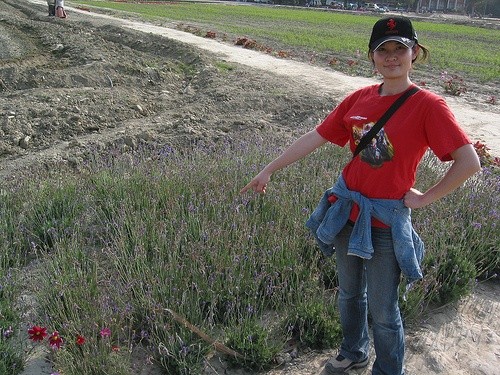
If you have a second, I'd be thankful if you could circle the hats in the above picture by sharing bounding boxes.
[369,16,417,53]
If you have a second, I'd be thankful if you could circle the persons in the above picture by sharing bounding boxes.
[47,0,67,18]
[239,15,480,375]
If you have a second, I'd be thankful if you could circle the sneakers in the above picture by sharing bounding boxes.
[325,354,369,375]
